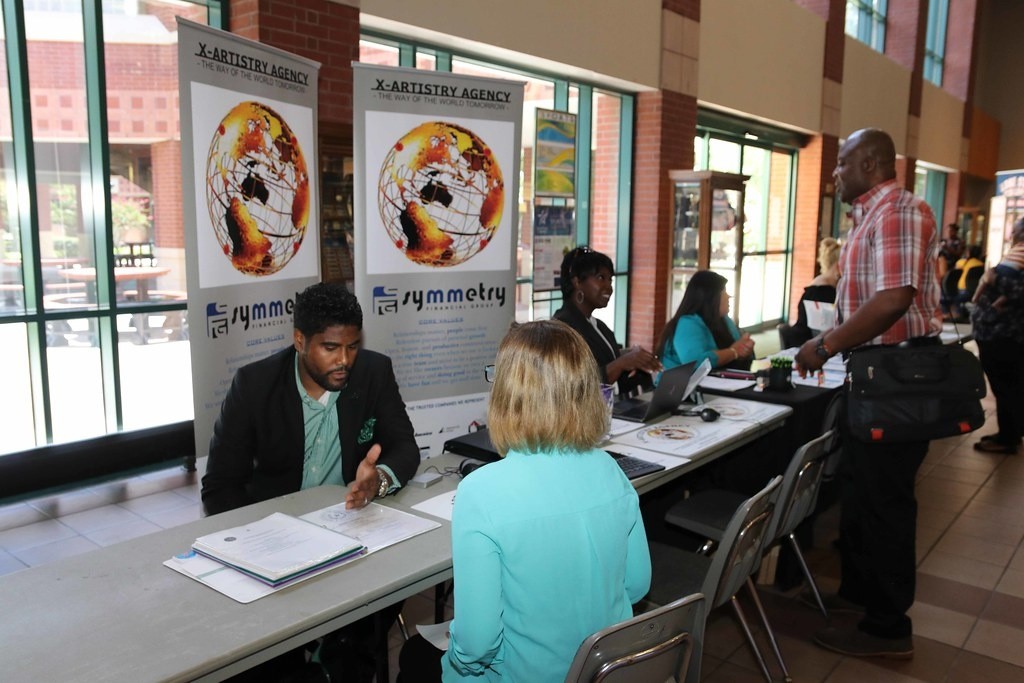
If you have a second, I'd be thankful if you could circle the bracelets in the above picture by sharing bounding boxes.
[730,347,739,359]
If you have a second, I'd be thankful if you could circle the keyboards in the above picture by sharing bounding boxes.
[615,456,665,479]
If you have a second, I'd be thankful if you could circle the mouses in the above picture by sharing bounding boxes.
[702,408,717,421]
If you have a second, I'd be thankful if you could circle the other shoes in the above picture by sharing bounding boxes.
[974,442,1018,454]
[800,590,868,616]
[811,625,914,661]
[980,435,1023,445]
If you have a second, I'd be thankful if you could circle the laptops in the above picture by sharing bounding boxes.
[610,357,713,422]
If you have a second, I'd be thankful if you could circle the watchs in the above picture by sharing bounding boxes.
[815,337,831,358]
[377,469,389,498]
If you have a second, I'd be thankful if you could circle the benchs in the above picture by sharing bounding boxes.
[0,280,189,347]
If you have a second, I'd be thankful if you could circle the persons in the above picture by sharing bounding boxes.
[938,223,984,309]
[202,281,421,683]
[794,127,943,659]
[971,215,1024,455]
[552,246,664,384]
[397,319,651,683]
[793,237,839,340]
[655,270,756,383]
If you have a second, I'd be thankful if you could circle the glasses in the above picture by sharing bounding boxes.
[569,245,596,274]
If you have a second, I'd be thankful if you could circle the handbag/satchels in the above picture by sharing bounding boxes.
[843,341,986,445]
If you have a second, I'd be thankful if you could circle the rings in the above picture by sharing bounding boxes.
[363,497,369,504]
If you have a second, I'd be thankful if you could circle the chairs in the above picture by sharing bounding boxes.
[937,265,984,336]
[820,390,856,489]
[646,475,793,683]
[667,431,835,621]
[563,593,704,683]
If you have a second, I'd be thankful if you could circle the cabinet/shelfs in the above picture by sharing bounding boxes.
[666,166,751,331]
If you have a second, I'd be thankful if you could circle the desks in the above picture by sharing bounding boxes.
[58,268,169,345]
[0,377,793,683]
[0,258,90,315]
[700,321,986,592]
